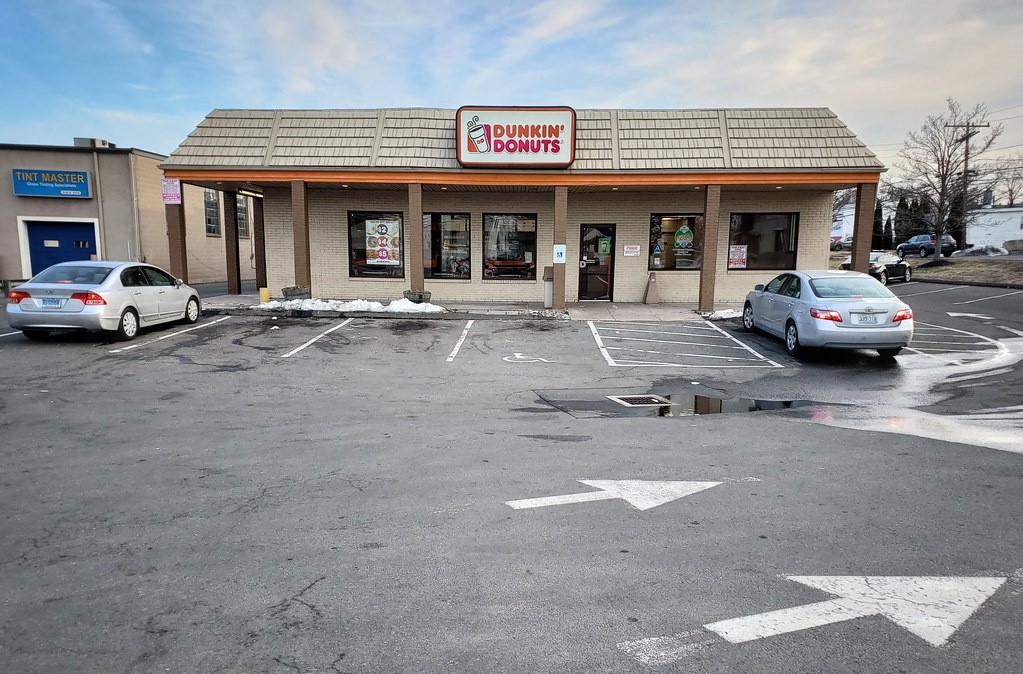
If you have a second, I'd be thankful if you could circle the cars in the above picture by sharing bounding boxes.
[4,258,201,339]
[830,235,853,251]
[896,234,957,258]
[742,269,914,359]
[839,251,913,285]
